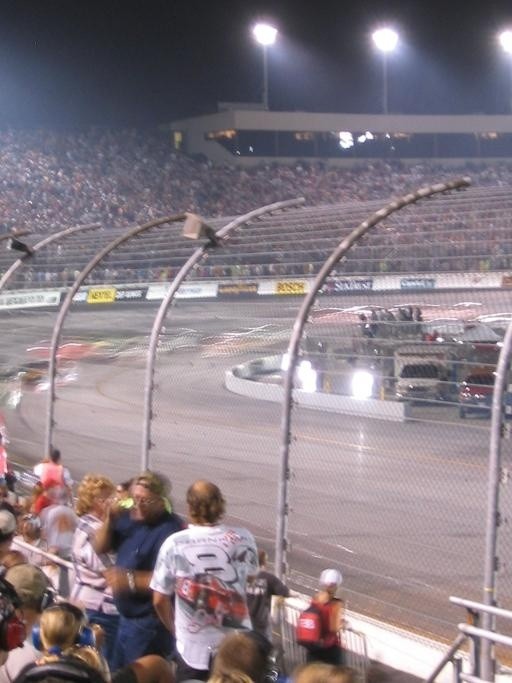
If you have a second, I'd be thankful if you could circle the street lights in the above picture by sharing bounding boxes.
[251,21,278,110]
[372,24,398,114]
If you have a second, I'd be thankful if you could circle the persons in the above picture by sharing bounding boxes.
[458,382,470,418]
[1,426,362,682]
[358,306,460,342]
[0,124,511,288]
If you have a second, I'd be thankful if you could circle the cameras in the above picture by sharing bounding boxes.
[38,589,54,613]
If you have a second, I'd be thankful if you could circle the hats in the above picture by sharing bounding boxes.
[133,470,165,495]
[319,568,342,588]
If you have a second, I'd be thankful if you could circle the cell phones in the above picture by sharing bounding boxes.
[118,498,134,511]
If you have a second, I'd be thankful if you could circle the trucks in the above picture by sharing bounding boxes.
[302,321,476,366]
[392,342,456,406]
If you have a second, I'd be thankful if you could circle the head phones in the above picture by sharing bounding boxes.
[207,629,285,682]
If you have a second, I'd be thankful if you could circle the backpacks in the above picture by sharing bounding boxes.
[295,602,337,653]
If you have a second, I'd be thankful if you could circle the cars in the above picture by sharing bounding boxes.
[418,318,504,348]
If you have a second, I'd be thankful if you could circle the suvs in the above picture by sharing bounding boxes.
[455,371,503,418]
